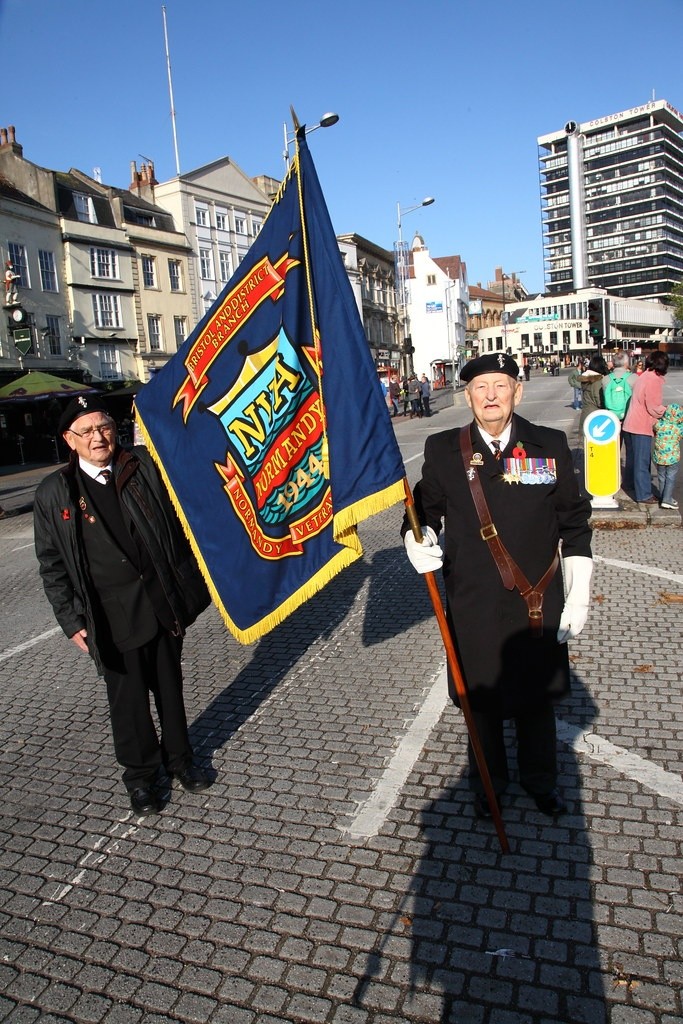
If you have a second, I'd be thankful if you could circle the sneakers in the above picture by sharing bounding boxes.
[661,499,680,509]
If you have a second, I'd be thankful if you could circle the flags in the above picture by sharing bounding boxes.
[133,123,407,642]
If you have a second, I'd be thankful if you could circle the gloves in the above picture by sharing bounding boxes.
[404,526,443,575]
[557,557,594,645]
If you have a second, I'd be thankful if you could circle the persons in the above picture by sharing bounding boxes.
[34,395,211,815]
[518,351,683,510]
[399,353,595,815]
[381,372,432,419]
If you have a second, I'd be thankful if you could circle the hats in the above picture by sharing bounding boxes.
[57,394,113,435]
[392,375,397,379]
[459,353,520,380]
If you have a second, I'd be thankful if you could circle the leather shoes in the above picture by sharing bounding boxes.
[176,763,207,791]
[131,786,159,816]
[519,781,566,815]
[474,793,501,822]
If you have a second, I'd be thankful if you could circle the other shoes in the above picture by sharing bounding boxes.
[637,495,661,503]
[574,406,581,410]
[394,413,431,419]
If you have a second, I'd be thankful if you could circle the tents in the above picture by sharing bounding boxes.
[0,371,145,411]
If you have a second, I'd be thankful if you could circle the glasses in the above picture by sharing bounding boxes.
[639,365,642,366]
[69,427,112,439]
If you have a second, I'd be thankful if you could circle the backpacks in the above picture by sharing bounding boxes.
[603,371,632,420]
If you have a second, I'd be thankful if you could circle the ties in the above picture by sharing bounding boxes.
[101,470,110,485]
[491,440,502,460]
